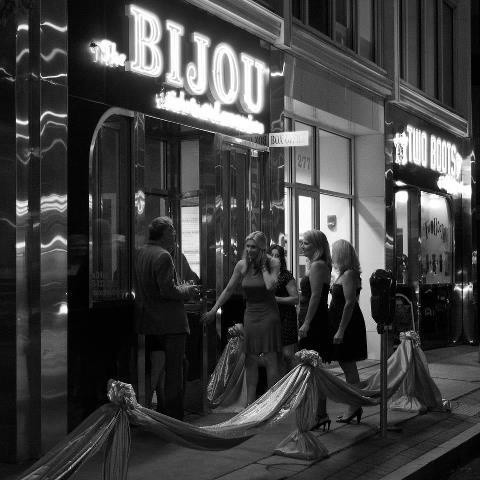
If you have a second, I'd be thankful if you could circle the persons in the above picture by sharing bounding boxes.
[329,239,368,424]
[199,231,299,410]
[131,216,199,419]
[299,230,332,432]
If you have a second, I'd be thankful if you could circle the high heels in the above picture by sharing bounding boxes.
[336,404,363,424]
[308,413,332,432]
